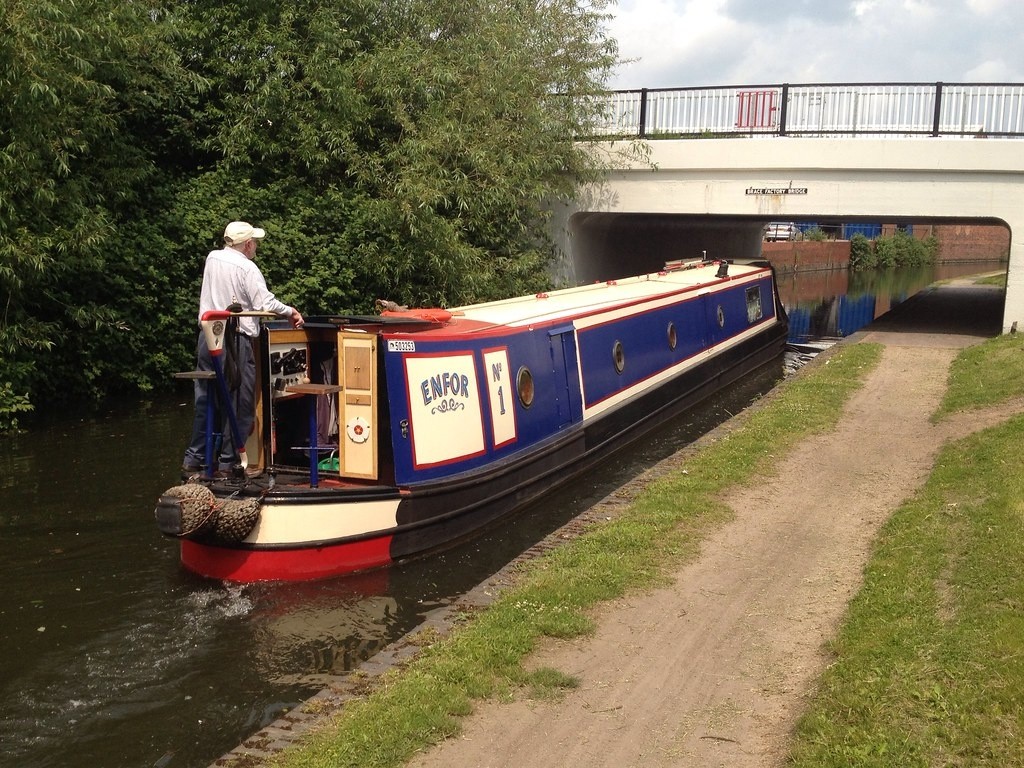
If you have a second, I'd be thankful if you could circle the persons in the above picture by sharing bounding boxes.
[182,221,304,476]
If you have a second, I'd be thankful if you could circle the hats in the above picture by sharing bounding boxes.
[224,221,267,246]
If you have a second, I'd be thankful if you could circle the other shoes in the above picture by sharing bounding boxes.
[200,467,231,481]
[183,464,198,471]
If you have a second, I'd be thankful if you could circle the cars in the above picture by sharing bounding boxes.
[766,222,798,242]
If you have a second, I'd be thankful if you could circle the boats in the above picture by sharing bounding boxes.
[181,260,794,582]
